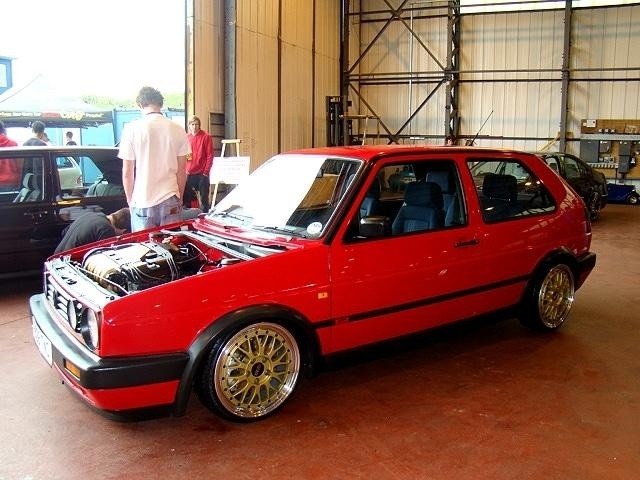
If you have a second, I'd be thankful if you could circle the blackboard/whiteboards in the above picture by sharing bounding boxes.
[209,156,251,185]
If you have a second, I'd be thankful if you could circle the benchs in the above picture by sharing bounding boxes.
[85,183,124,197]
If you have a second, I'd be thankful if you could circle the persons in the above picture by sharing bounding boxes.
[183,116,214,214]
[51,206,132,259]
[41,132,50,146]
[24,121,50,146]
[118,83,191,238]
[63,129,81,166]
[0,122,22,192]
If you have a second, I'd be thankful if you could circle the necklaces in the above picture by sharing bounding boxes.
[146,112,163,116]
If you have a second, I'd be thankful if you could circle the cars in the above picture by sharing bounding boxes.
[0,145,201,283]
[56,156,83,189]
[28,144,597,424]
[469,150,608,224]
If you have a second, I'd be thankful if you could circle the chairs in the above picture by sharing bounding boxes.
[426,169,460,225]
[12,172,35,203]
[343,174,380,218]
[482,173,518,220]
[391,180,446,238]
[26,175,48,202]
